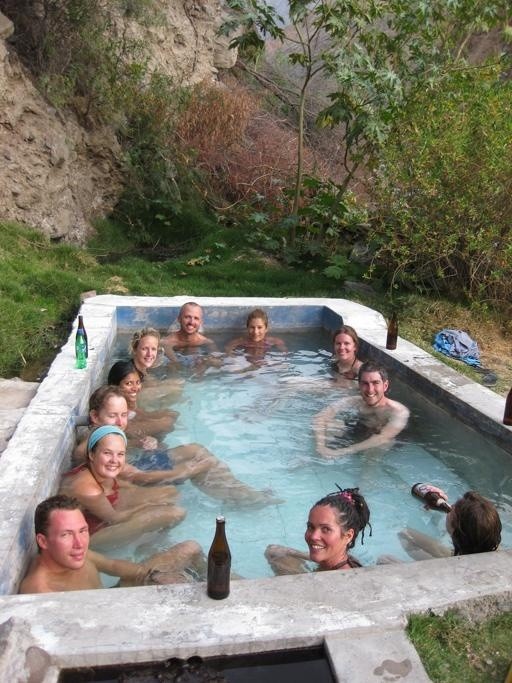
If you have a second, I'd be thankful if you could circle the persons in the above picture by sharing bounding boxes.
[264,484,373,577]
[60,426,187,553]
[313,360,410,460]
[18,495,245,595]
[160,302,222,371]
[127,327,185,409]
[107,359,180,441]
[375,484,503,565]
[225,309,289,375]
[285,325,366,395]
[73,384,286,510]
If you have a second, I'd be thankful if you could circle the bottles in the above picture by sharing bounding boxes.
[385,311,398,349]
[76,315,89,358]
[75,328,87,367]
[502,387,512,425]
[411,482,451,514]
[206,517,231,600]
